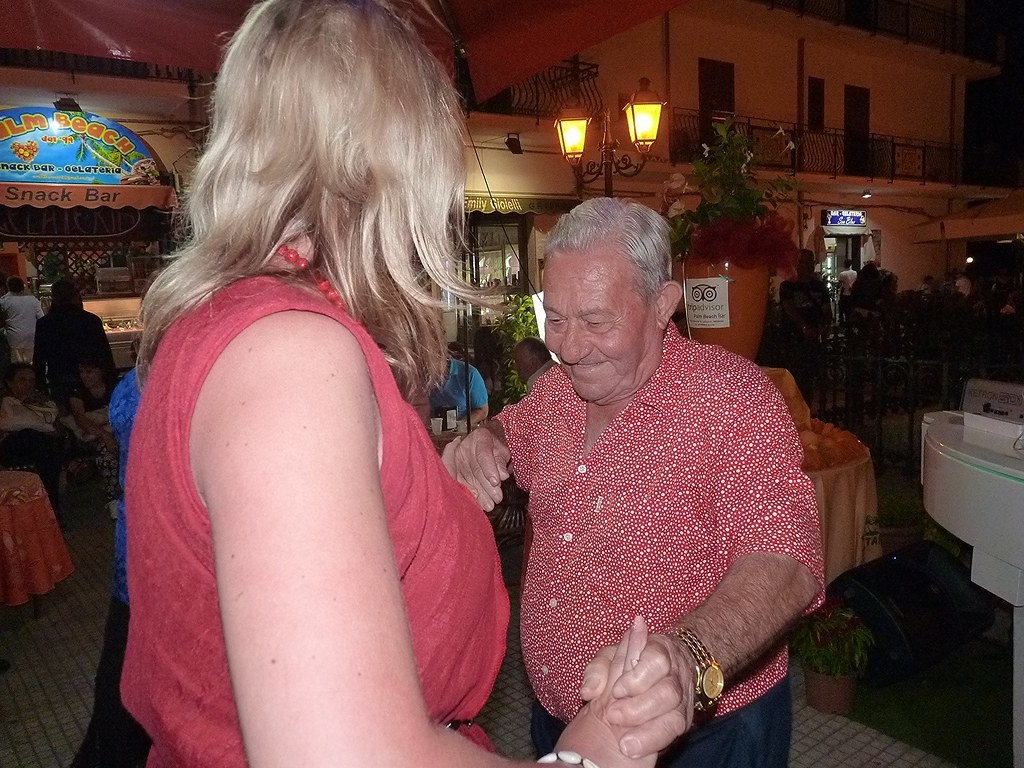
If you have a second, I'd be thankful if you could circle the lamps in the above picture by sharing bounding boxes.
[502,133,523,155]
[861,189,872,199]
[53,90,84,115]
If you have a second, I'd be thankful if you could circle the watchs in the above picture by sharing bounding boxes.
[659,628,725,711]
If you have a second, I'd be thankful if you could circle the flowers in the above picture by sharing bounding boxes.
[789,600,874,673]
[657,119,796,263]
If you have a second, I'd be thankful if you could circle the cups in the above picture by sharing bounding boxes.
[430,417,443,436]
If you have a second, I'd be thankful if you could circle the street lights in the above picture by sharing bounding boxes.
[552,77,668,200]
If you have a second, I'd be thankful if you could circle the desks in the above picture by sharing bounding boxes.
[0,470,75,605]
[428,433,527,542]
[759,367,882,591]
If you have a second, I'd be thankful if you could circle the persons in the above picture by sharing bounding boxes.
[120,0,658,768]
[514,337,557,395]
[780,249,1024,431]
[0,272,153,768]
[456,197,825,768]
[427,337,489,424]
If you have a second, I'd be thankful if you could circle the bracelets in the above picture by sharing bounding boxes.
[537,751,598,768]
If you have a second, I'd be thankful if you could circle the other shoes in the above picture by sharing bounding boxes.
[104,499,118,519]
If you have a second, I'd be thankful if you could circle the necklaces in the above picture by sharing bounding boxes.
[275,242,346,310]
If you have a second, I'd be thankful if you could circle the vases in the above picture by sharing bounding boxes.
[803,669,855,716]
[682,230,770,365]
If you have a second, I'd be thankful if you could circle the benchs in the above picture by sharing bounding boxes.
[11,367,136,465]
